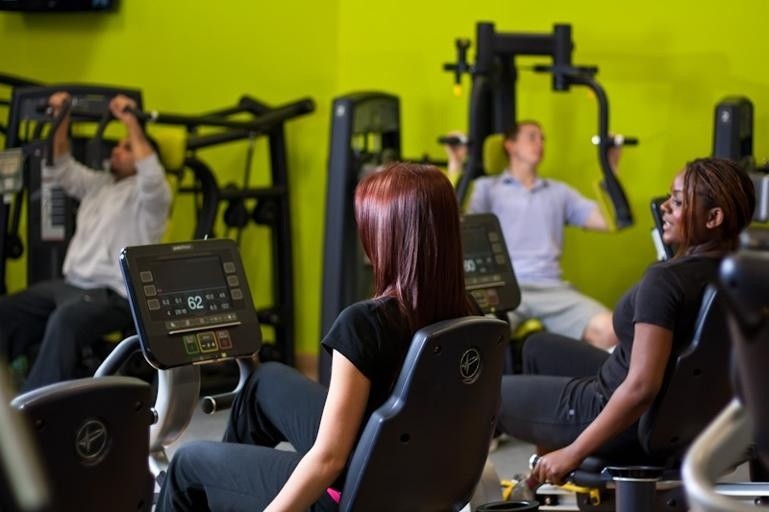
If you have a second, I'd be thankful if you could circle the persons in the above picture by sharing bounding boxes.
[0,90,172,394]
[445,119,623,351]
[495,155,757,486]
[153,159,486,512]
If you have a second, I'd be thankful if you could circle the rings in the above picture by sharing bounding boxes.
[544,479,552,483]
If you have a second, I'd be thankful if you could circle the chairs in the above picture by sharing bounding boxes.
[0,371,157,508]
[321,310,516,510]
[584,242,769,512]
